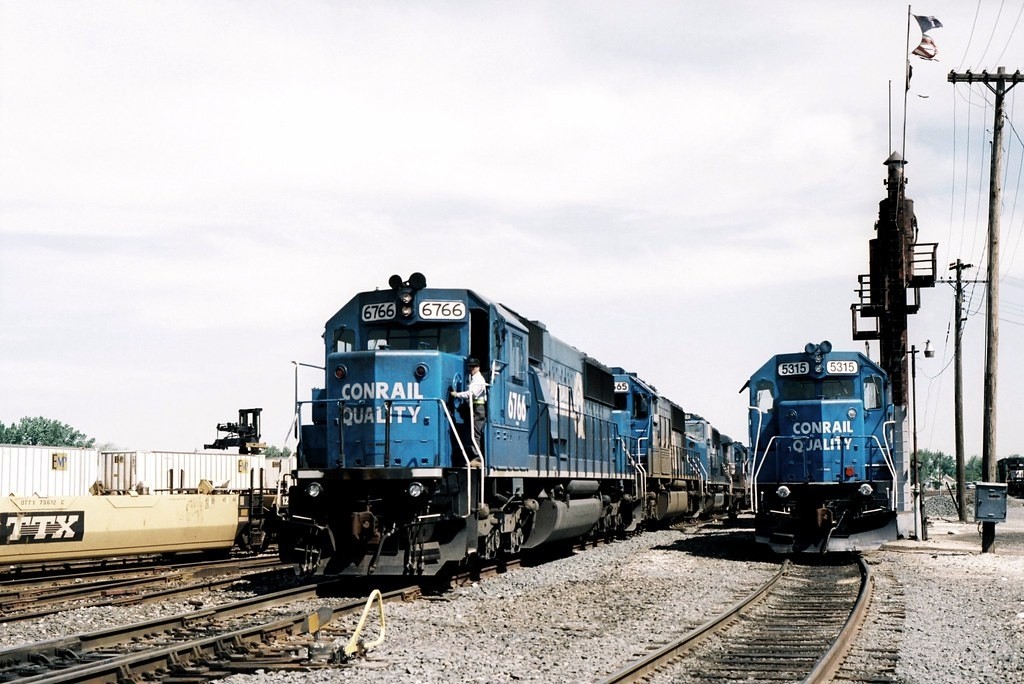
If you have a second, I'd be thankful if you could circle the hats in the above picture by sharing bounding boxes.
[464,359,480,365]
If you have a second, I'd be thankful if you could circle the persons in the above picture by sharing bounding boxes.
[450,357,487,467]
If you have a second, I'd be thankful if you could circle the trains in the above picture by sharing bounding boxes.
[738,341,899,556]
[995,454,1024,498]
[276,271,751,588]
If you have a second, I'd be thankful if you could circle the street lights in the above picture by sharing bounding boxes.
[906,340,935,541]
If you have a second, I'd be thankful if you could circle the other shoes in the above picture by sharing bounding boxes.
[465,460,481,467]
